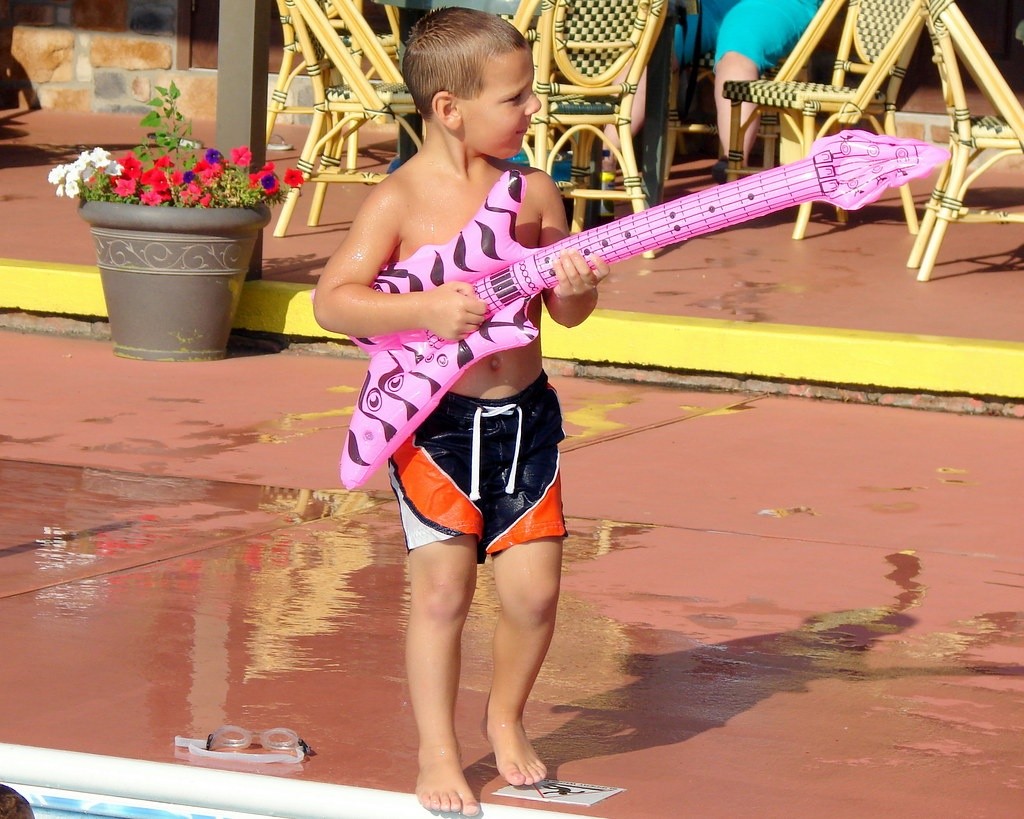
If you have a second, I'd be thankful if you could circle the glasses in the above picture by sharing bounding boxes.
[175,725,313,763]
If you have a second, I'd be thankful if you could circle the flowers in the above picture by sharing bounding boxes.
[47,79,303,209]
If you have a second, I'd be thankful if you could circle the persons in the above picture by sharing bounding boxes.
[578,0,825,217]
[313,7,611,818]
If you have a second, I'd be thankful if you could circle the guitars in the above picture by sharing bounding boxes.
[338,128,952,493]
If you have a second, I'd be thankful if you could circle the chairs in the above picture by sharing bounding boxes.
[907,0,1024,283]
[662,0,924,238]
[499,0,668,258]
[264,0,419,236]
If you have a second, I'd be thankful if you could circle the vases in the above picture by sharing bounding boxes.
[79,202,272,363]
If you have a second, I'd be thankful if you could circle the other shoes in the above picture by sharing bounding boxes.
[710,159,729,182]
